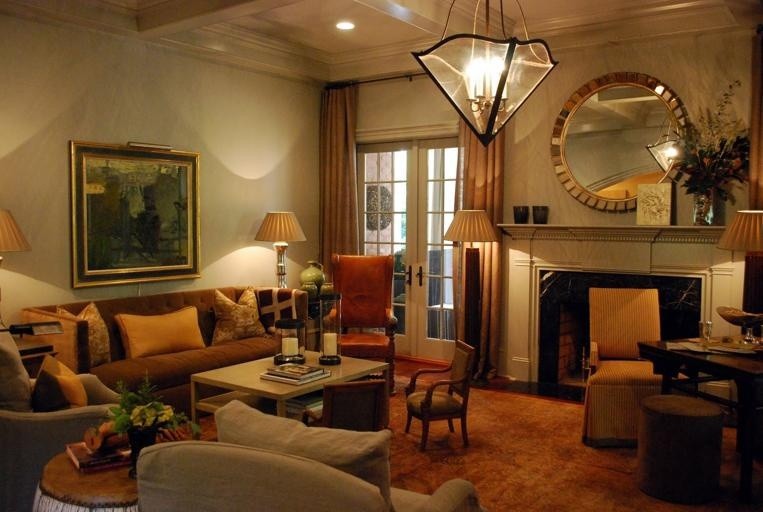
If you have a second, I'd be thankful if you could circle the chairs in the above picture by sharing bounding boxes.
[301,383,388,433]
[322,255,396,394]
[581,287,689,449]
[404,342,477,451]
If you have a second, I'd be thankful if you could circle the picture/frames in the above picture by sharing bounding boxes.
[68,138,203,288]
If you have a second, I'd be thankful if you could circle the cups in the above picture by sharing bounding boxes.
[533,206,548,225]
[514,206,529,224]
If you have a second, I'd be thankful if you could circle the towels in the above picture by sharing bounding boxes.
[665,341,709,353]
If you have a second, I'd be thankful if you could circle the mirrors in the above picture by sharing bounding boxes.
[549,73,692,212]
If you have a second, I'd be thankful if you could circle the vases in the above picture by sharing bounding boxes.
[300,260,325,289]
[302,282,318,301]
[693,189,714,227]
[320,283,333,298]
[127,428,169,477]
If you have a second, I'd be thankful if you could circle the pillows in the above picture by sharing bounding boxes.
[212,288,264,346]
[210,400,392,509]
[77,302,110,367]
[30,355,89,411]
[114,306,207,358]
[55,305,90,376]
[0,320,32,412]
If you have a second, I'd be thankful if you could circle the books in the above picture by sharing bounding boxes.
[66,441,131,474]
[260,363,332,386]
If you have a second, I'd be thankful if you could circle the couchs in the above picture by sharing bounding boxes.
[22,286,310,419]
[136,443,482,512]
[0,373,120,511]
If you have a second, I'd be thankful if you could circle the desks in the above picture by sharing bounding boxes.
[35,452,138,512]
[633,334,763,505]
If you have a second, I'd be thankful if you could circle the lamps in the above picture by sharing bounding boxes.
[0,209,32,330]
[442,211,499,385]
[336,21,355,31]
[713,212,763,253]
[254,212,306,289]
[409,1,558,147]
[645,115,679,181]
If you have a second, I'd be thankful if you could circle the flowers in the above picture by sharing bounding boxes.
[108,372,202,433]
[669,82,750,204]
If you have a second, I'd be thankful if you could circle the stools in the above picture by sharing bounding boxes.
[637,396,724,503]
